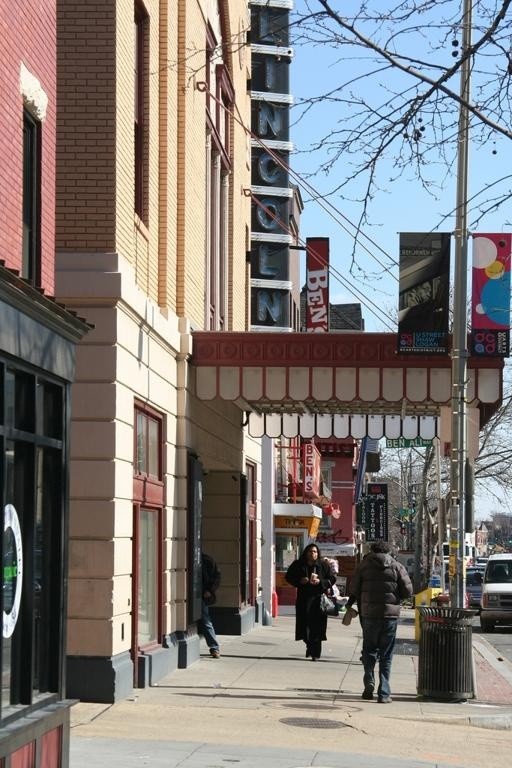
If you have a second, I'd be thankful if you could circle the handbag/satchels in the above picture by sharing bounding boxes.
[321,593,339,616]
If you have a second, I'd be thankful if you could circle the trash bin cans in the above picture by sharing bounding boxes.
[415,605,478,703]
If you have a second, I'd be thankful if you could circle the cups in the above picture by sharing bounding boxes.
[309,573,319,584]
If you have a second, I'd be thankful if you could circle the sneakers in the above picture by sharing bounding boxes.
[377,697,393,703]
[213,649,221,658]
[363,683,374,699]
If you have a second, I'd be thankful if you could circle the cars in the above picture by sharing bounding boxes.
[465,557,488,615]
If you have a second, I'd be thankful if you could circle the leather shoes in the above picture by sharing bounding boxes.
[306,648,319,661]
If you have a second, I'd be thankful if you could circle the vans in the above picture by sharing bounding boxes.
[479,552,512,632]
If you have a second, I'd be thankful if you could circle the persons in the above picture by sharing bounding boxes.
[199,552,221,658]
[348,541,413,703]
[285,544,337,661]
